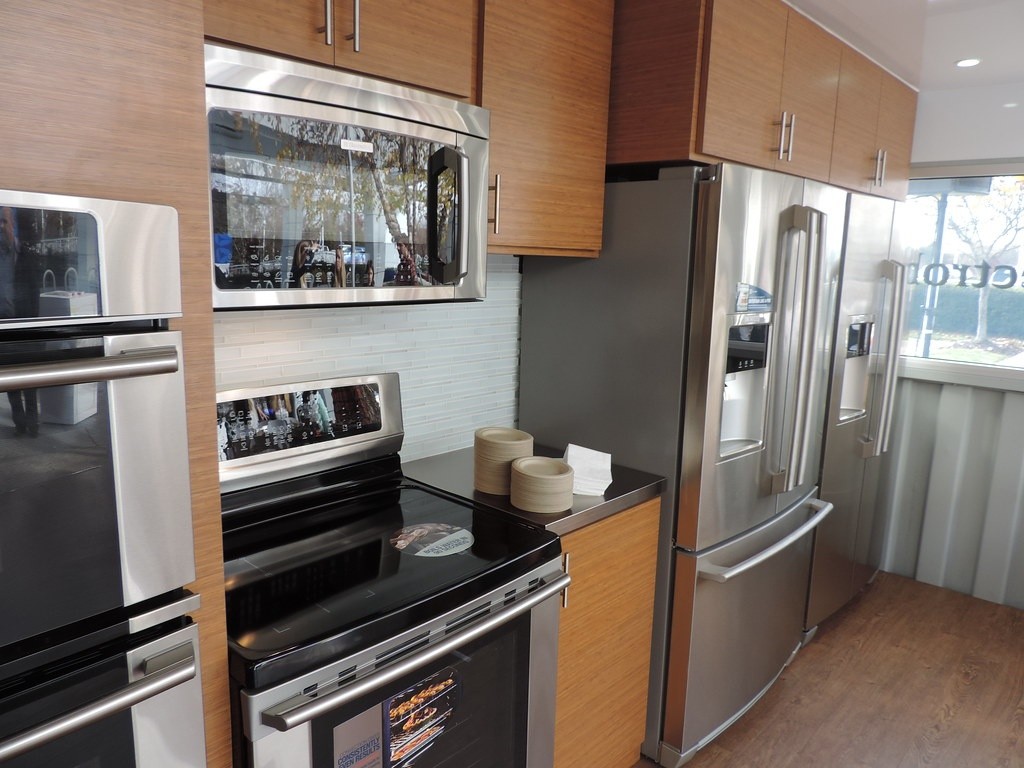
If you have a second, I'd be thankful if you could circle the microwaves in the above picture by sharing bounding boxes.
[204,38,497,313]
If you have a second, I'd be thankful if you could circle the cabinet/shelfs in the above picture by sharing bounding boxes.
[203,0,921,258]
[552,496,666,768]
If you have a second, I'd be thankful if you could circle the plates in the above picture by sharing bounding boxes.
[474,425,573,513]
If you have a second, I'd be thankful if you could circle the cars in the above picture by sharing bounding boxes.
[332,245,366,272]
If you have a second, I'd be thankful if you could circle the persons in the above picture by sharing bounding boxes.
[0,206,39,438]
[366,260,375,286]
[392,233,417,285]
[336,247,345,287]
[291,240,314,288]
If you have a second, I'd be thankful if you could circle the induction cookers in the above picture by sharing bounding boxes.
[215,365,564,696]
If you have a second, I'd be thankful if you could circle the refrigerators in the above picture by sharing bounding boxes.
[516,162,910,768]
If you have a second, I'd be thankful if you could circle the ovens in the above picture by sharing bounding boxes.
[0,187,208,768]
[223,553,568,768]
